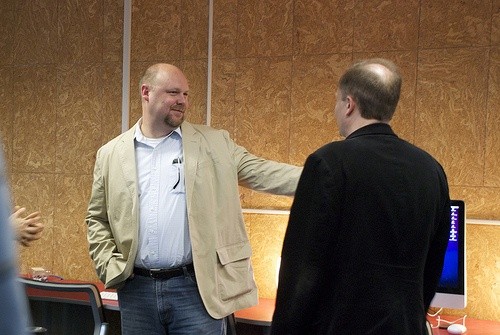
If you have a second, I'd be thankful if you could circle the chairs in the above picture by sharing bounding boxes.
[15,278,110,335]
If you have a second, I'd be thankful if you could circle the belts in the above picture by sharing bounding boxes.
[131,262,194,278]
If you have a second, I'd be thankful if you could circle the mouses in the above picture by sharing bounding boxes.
[447,324,466,334]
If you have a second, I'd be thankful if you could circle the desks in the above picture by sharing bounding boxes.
[19,274,500,335]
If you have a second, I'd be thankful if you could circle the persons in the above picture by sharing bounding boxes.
[270,56,451,335]
[0,150,34,335]
[85,63,305,335]
[10,205,43,249]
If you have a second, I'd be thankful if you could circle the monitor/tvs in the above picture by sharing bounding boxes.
[429,199,467,309]
